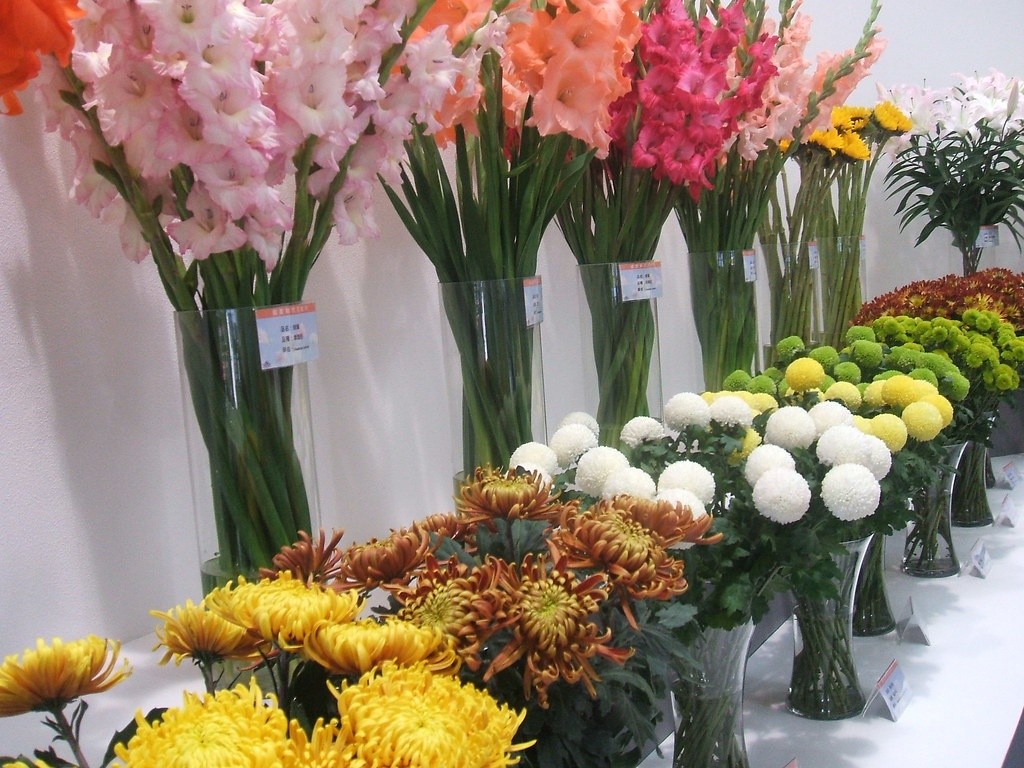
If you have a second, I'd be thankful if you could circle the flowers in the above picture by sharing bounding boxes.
[1,0,912,564]
[873,64,1024,258]
[0,268,1024,768]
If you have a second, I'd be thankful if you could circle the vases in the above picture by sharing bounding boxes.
[986,386,1024,457]
[576,263,665,445]
[812,234,871,348]
[662,580,755,768]
[951,410,995,527]
[749,242,826,369]
[783,532,877,721]
[433,276,548,509]
[682,251,763,393]
[948,224,1001,278]
[172,302,326,696]
[899,443,966,577]
[853,523,896,638]
